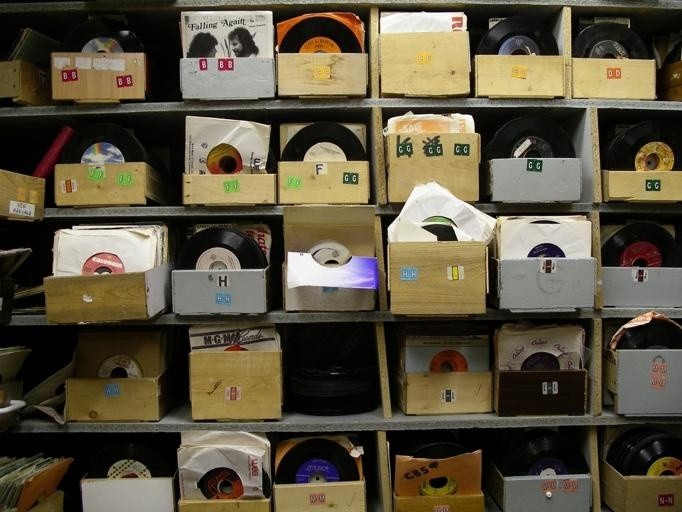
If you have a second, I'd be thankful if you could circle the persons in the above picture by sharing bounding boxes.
[186,32,218,58]
[228,26,259,58]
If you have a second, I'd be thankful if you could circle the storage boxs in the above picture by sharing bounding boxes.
[1,0,682,510]
